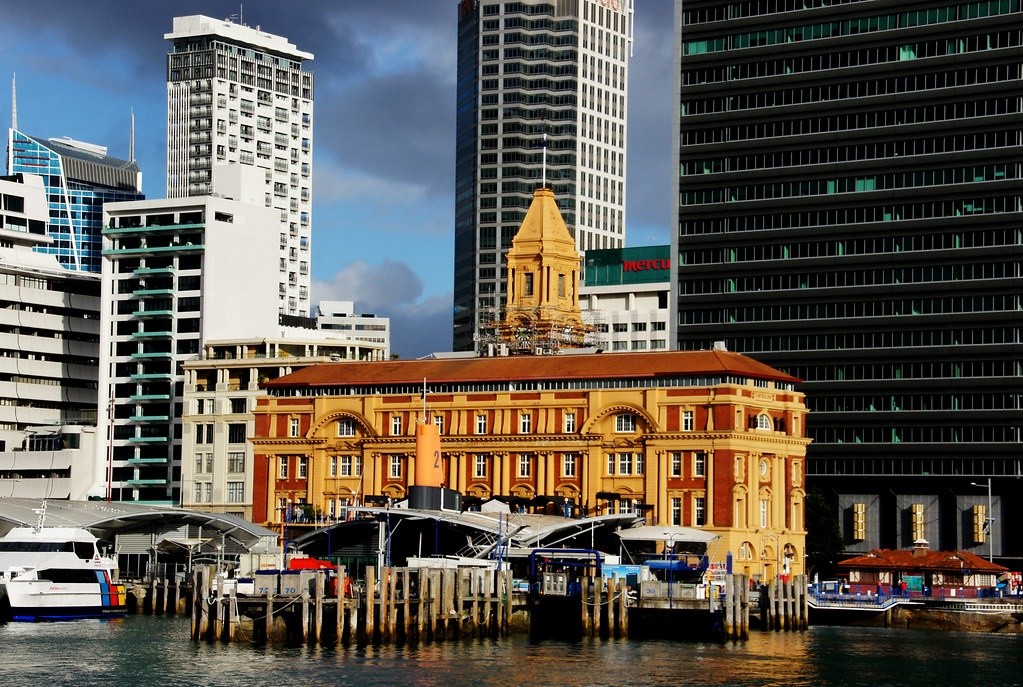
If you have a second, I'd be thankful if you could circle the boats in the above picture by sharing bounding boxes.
[0,498,130,622]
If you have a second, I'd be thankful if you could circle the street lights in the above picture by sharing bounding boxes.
[970,478,992,563]
[663,532,685,609]
